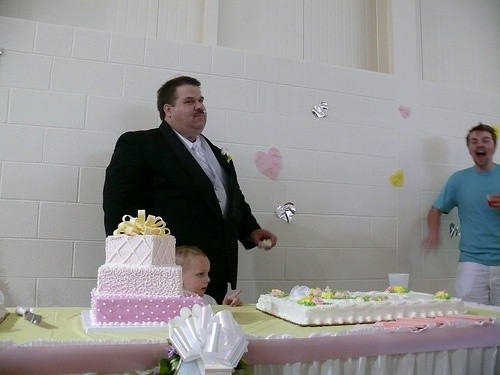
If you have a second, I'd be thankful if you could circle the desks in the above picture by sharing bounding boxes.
[0,304,500,375]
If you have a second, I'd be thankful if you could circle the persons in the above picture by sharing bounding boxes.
[420,124,500,307]
[175,246,244,309]
[102,75,278,304]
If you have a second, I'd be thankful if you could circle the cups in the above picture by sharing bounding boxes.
[388,273,409,289]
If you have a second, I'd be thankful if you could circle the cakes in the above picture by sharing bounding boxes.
[255,285,465,325]
[90,209,204,327]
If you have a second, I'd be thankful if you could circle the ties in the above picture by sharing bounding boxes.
[192,145,216,183]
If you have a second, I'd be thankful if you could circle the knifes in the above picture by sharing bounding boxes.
[15,305,42,325]
